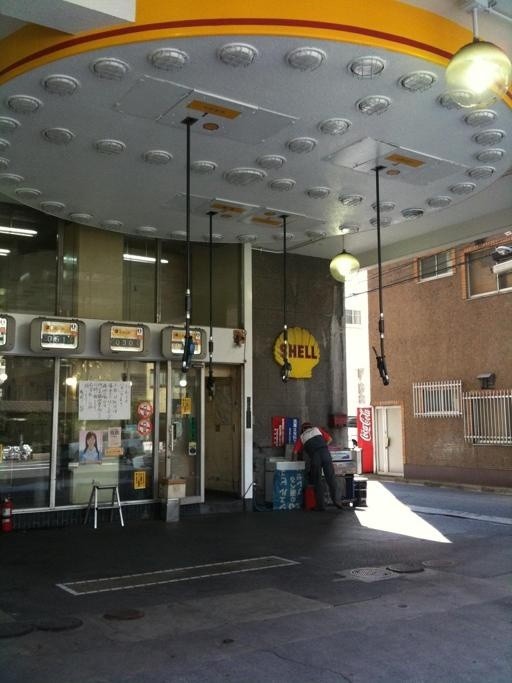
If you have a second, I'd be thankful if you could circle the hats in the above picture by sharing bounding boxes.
[301,423,313,431]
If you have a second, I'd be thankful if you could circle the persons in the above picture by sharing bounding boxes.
[80,431,100,461]
[292,422,347,511]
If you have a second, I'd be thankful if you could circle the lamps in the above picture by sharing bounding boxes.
[330,235,360,283]
[445,7,512,110]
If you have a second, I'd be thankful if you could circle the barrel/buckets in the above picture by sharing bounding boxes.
[305,483,316,509]
[354,482,367,507]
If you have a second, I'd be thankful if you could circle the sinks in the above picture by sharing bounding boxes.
[1,463,49,480]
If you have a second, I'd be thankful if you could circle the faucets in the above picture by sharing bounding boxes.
[19,433,26,460]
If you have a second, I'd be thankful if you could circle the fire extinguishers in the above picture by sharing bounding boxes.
[1,495,13,533]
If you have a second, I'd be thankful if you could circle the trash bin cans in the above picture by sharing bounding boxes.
[353,478,367,506]
